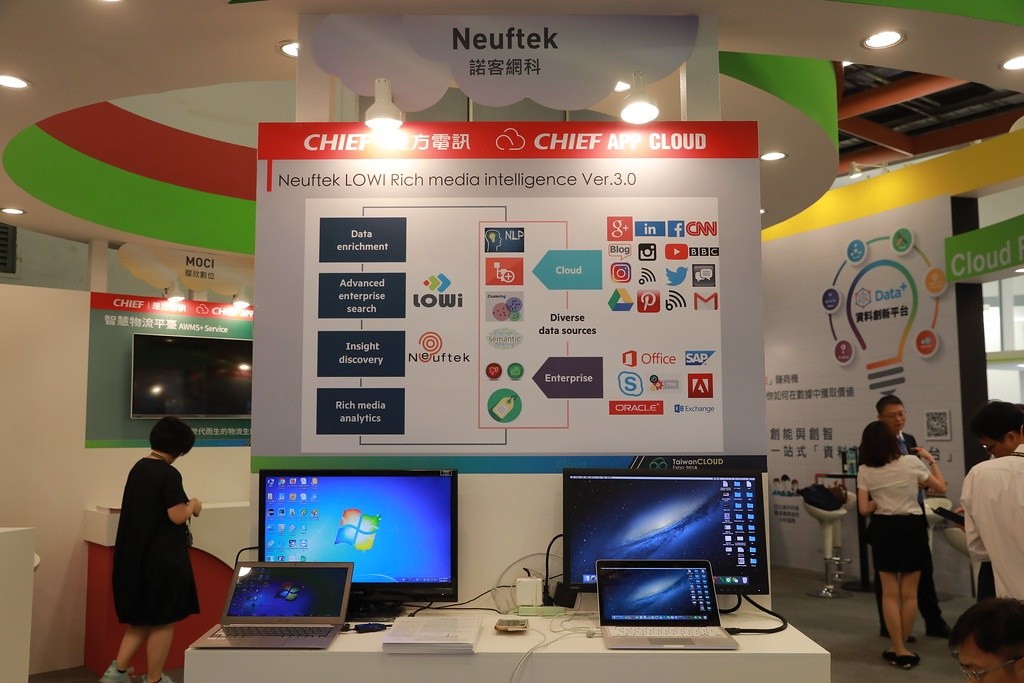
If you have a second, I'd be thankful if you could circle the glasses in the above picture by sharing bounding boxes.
[982,434,1008,452]
[881,409,908,418]
[960,655,1024,682]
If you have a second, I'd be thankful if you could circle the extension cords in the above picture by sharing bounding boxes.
[518,605,566,616]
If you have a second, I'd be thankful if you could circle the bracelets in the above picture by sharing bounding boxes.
[929,460,937,465]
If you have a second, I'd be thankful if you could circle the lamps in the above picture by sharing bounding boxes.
[233,294,250,310]
[165,280,185,303]
[364,77,405,134]
[619,71,661,125]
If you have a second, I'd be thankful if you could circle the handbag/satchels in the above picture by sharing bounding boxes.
[796,483,847,510]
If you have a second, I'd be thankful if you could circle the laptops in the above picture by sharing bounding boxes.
[595,558,740,650]
[190,562,354,649]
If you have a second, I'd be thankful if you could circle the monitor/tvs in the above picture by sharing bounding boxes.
[131,333,254,419]
[561,467,770,596]
[258,469,458,623]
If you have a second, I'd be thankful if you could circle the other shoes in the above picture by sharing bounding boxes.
[926,625,952,639]
[882,650,897,665]
[897,653,920,670]
[880,629,916,644]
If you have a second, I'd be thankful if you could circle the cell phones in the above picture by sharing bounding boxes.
[494,619,528,631]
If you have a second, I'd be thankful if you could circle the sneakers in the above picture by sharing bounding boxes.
[141,672,173,683]
[98,660,134,683]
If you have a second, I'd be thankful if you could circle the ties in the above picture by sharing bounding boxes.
[898,436,909,455]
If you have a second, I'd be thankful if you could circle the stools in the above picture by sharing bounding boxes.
[922,497,978,617]
[802,490,861,599]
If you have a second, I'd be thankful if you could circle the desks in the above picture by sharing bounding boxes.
[828,472,882,593]
[182,602,831,683]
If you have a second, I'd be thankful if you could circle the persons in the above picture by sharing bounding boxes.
[961,420,1024,600]
[858,421,946,668]
[954,402,1022,601]
[97,415,202,683]
[949,598,1024,683]
[876,395,952,642]
